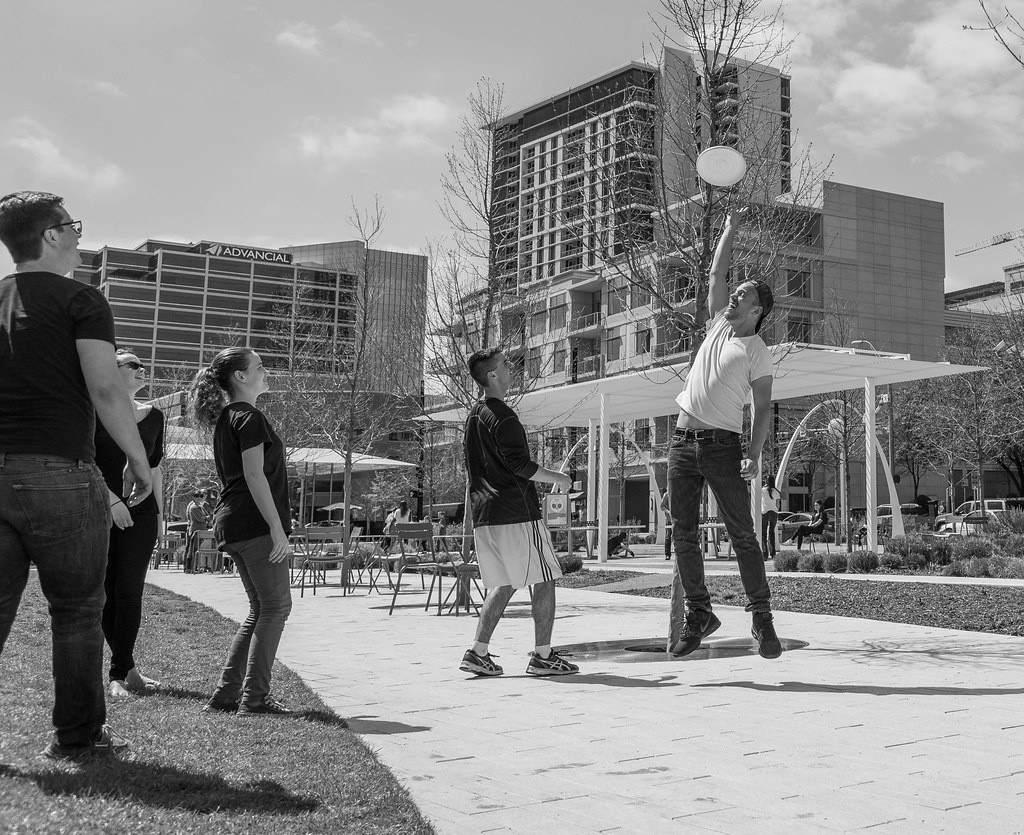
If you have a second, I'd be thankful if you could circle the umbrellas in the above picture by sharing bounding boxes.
[317,502,363,526]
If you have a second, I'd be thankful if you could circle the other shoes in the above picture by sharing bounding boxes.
[782,539,792,546]
[666,555,670,559]
[611,554,621,558]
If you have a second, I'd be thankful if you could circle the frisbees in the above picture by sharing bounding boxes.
[695,145,747,187]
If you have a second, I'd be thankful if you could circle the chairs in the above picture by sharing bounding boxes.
[809,518,831,554]
[150,517,486,619]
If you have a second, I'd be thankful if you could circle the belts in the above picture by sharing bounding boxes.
[675,428,732,440]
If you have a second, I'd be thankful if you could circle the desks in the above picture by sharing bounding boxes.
[665,523,733,561]
[571,525,647,560]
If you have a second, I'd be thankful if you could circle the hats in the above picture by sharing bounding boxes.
[750,280,774,334]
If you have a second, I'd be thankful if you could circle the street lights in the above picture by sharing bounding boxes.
[852,339,896,482]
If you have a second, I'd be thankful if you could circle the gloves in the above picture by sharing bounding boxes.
[631,552,634,557]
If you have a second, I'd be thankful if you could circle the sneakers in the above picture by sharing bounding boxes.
[751,612,782,659]
[526,648,579,674]
[203,697,238,714]
[237,693,294,716]
[459,649,503,675]
[672,611,721,657]
[45,724,130,757]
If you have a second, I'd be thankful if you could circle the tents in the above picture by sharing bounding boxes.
[162,443,418,529]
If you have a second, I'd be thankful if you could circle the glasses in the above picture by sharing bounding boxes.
[50,220,82,234]
[194,494,203,498]
[118,362,145,370]
[814,505,818,506]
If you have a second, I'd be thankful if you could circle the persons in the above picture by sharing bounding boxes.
[660,491,675,560]
[383,500,450,552]
[459,345,579,676]
[187,344,292,715]
[608,530,635,559]
[761,475,827,561]
[666,206,785,660]
[184,489,212,574]
[0,191,166,761]
[570,504,587,541]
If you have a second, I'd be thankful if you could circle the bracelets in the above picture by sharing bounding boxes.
[111,500,121,507]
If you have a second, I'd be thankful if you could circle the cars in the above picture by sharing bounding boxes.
[776,498,1024,538]
